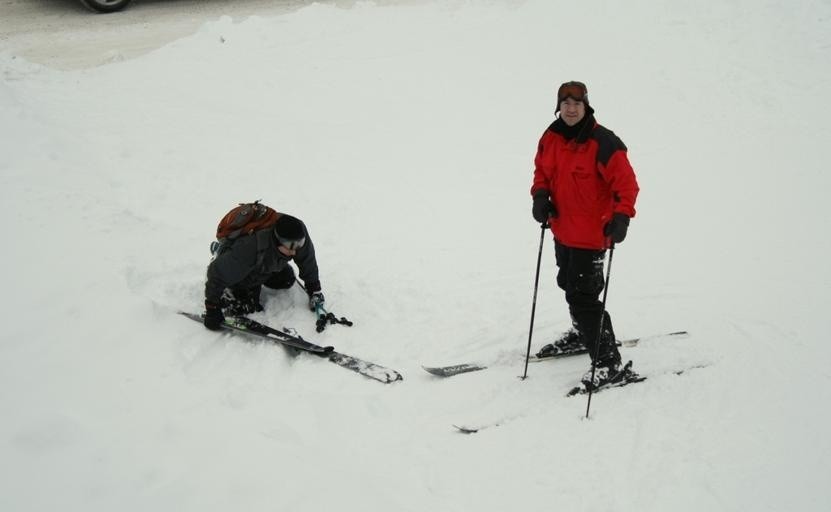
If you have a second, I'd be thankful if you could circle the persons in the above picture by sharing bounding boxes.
[200,202,326,330]
[529,79,640,390]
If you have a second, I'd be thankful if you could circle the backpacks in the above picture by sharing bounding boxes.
[216,203,280,240]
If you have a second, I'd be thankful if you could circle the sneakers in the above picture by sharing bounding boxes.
[582,356,624,390]
[554,326,585,351]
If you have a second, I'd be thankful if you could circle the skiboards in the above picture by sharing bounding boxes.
[420,331,722,432]
[175,305,403,383]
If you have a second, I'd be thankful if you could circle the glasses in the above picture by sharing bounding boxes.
[558,85,585,101]
[281,237,305,249]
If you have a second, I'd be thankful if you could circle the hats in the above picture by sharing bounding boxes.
[558,81,588,103]
[273,214,307,250]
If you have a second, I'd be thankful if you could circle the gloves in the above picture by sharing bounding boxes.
[604,212,629,242]
[531,188,558,222]
[203,299,224,334]
[310,291,323,313]
